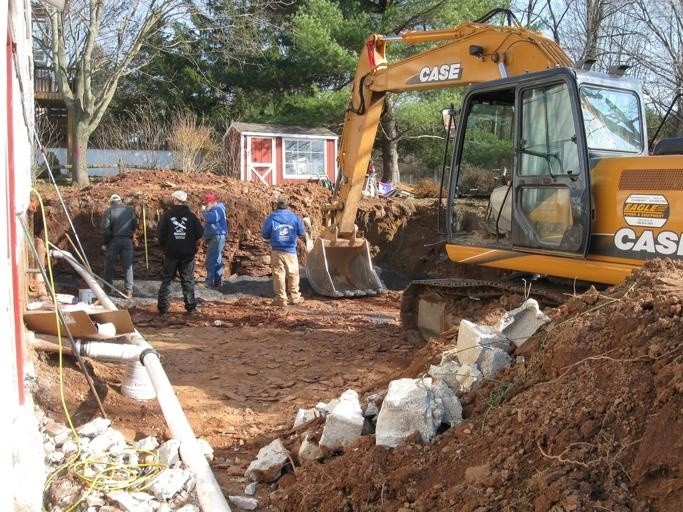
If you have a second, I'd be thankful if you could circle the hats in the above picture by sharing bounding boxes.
[202,194,216,203]
[277,194,290,203]
[171,190,188,201]
[108,194,121,201]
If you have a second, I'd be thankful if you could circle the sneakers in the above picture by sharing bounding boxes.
[269,296,304,307]
[124,292,133,299]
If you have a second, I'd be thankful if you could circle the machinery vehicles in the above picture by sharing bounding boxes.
[303,7,683,346]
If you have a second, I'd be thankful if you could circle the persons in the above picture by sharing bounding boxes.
[34,143,63,180]
[198,192,229,290]
[155,189,205,317]
[259,193,306,308]
[25,193,58,298]
[98,193,137,301]
[487,176,512,238]
